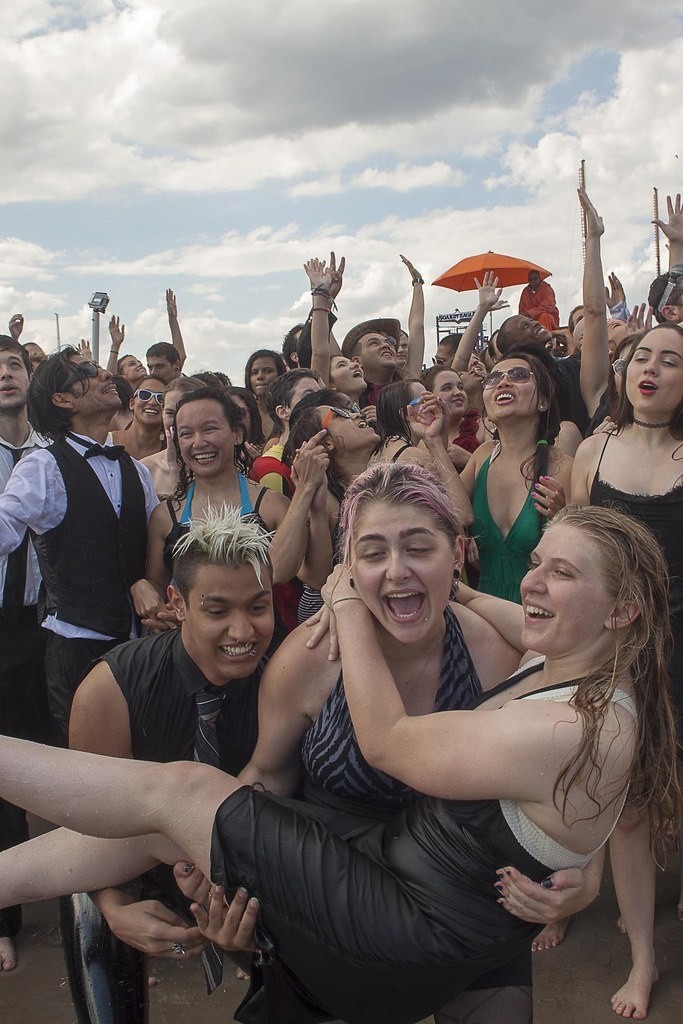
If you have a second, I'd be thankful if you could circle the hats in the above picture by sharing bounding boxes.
[342,319,401,359]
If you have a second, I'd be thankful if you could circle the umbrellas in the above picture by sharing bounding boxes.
[430,250,552,338]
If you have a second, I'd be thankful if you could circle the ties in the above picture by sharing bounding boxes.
[194,685,223,996]
[0,442,30,627]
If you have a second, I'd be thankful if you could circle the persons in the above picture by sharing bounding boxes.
[532,184,683,951]
[0,289,187,973]
[567,321,682,1019]
[143,247,583,607]
[0,504,683,1023]
[172,462,606,1023]
[56,497,278,1024]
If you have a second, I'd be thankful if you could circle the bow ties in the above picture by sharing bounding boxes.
[68,432,125,461]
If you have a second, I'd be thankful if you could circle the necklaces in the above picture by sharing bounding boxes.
[631,418,673,430]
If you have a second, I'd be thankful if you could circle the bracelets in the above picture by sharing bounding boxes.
[110,350,119,354]
[331,595,363,609]
[311,287,330,293]
[311,306,331,313]
[311,291,339,311]
[412,278,425,286]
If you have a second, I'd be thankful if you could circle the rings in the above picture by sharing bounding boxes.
[173,942,187,956]
[582,868,600,903]
[207,891,213,898]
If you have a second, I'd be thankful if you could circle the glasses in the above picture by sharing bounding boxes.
[322,402,366,429]
[387,335,396,346]
[60,361,102,393]
[658,264,683,312]
[480,366,535,389]
[432,356,452,367]
[133,388,163,406]
[528,277,539,281]
[611,359,626,378]
